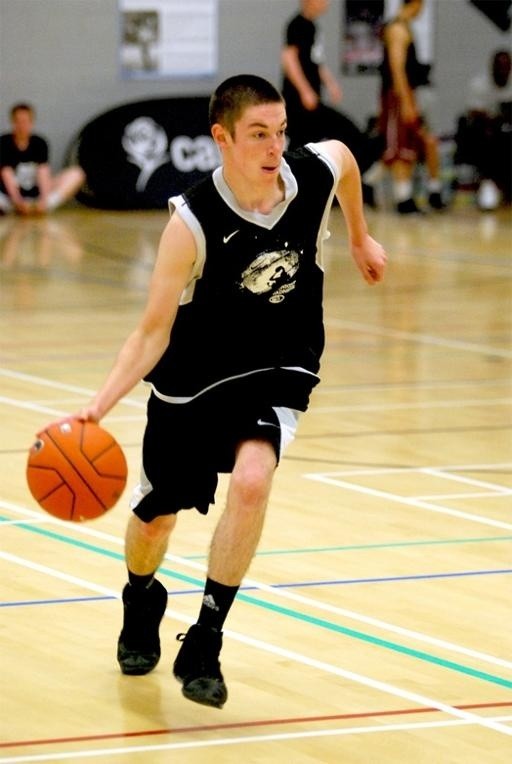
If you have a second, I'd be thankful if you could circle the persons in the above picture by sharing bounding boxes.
[0,218,85,314]
[0,100,87,221]
[66,70,391,712]
[280,2,512,217]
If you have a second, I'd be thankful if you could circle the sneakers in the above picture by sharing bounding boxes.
[116,575,171,676]
[171,623,232,706]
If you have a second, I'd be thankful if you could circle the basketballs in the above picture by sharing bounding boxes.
[27,414,127,523]
